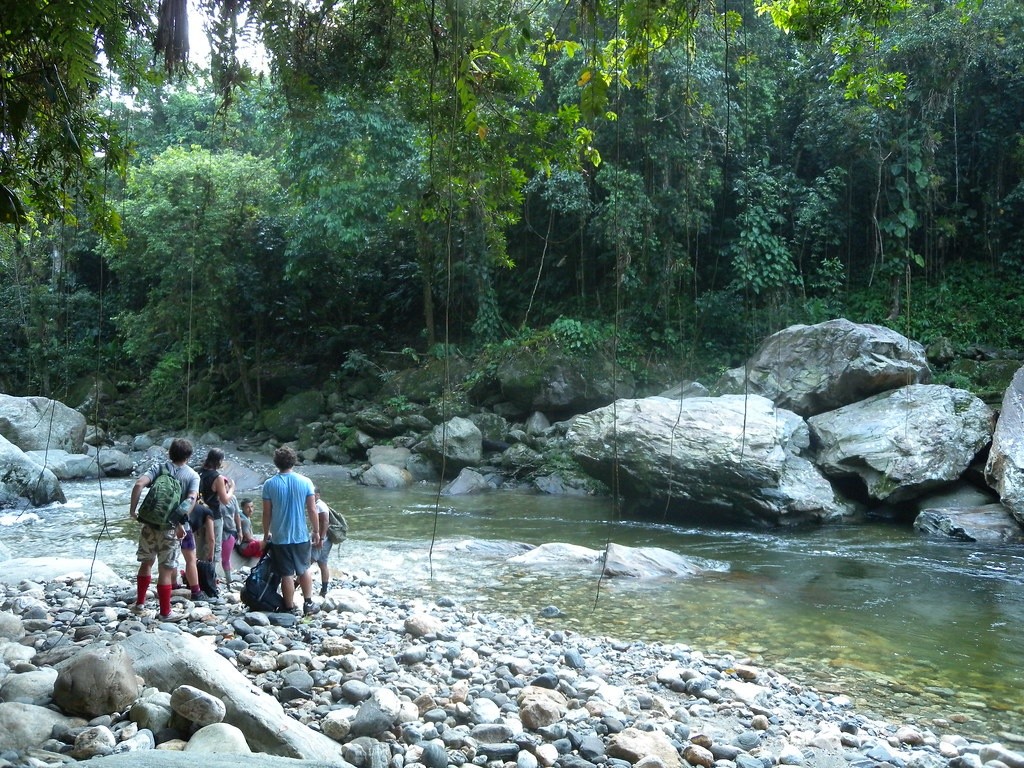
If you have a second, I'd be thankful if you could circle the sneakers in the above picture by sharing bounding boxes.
[131,604,145,614]
[317,596,326,605]
[285,602,302,616]
[191,591,216,602]
[159,609,188,622]
[303,601,320,614]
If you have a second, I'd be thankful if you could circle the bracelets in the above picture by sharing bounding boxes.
[313,530,320,534]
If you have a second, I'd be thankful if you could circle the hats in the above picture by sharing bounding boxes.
[223,476,229,484]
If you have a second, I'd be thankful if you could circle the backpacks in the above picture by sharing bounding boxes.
[241,545,285,613]
[137,464,195,531]
[196,467,217,508]
[316,502,348,544]
[185,560,220,599]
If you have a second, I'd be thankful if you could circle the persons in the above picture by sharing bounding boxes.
[234,498,267,558]
[262,448,322,614]
[130,437,243,622]
[293,485,332,598]
